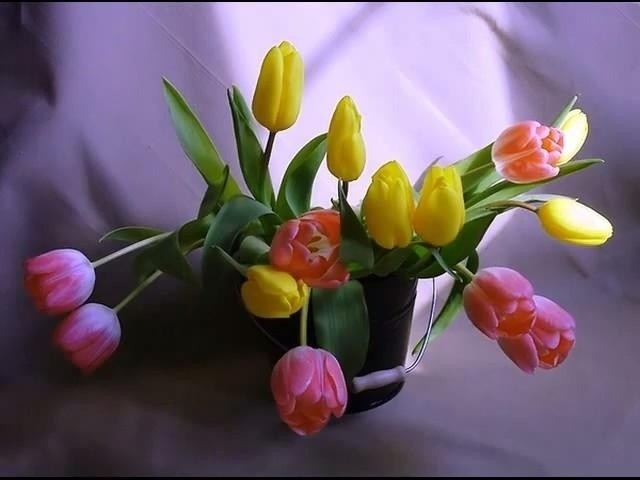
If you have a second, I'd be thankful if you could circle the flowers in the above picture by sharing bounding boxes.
[20,39,614,437]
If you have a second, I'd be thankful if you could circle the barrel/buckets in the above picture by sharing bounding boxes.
[241,268,438,417]
